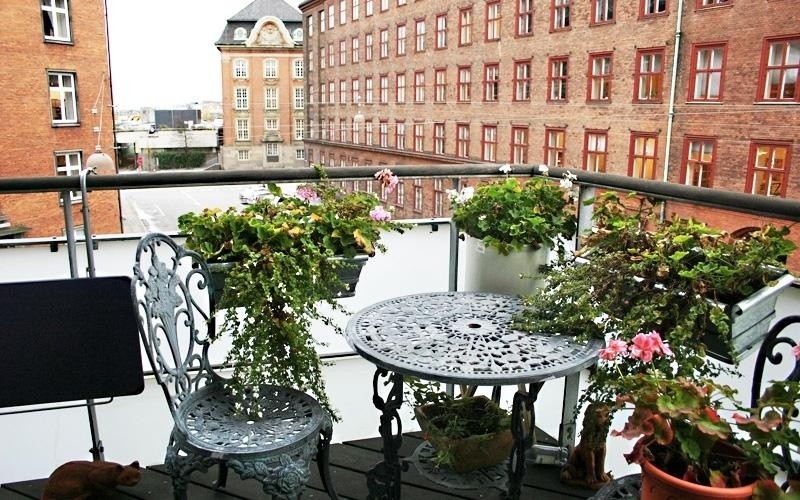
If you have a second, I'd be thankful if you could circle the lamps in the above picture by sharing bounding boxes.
[130,231,340,499]
[587,314,800,499]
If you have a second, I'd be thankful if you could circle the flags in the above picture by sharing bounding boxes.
[455,230,551,300]
[632,438,765,500]
[195,252,367,310]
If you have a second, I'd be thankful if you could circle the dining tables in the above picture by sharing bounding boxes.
[507,196,800,365]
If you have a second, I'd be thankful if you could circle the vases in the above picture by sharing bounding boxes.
[598,333,800,500]
[443,162,579,247]
[176,163,416,414]
